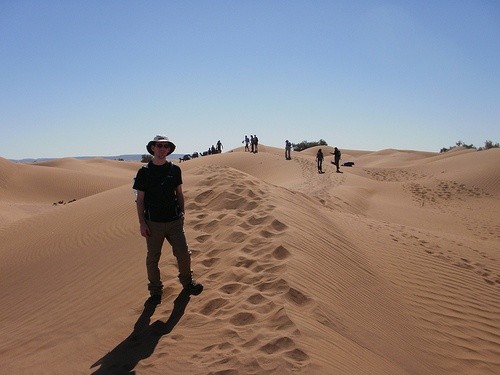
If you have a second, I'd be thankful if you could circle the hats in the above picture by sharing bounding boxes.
[146,135,176,156]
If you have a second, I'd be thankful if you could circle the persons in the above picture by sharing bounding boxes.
[215,140,223,154]
[250,135,258,153]
[330,146,341,172]
[285,140,291,160]
[315,149,324,174]
[242,136,250,152]
[208,145,215,155]
[131,135,203,303]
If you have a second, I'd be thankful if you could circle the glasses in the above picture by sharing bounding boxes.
[155,144,170,149]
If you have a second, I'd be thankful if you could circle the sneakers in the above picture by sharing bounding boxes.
[183,280,204,292]
[149,288,163,297]
